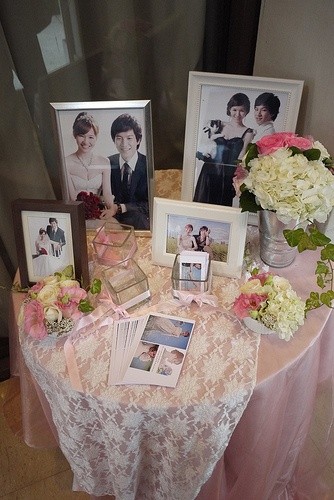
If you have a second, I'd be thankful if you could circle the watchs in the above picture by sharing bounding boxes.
[115,203,122,215]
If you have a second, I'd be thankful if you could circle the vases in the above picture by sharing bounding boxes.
[242,317,276,335]
[257,208,302,268]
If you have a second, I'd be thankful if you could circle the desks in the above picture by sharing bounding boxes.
[8,169,334,500]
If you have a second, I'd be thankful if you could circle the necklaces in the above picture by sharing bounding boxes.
[77,152,93,181]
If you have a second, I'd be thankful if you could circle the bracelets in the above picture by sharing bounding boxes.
[206,236,209,237]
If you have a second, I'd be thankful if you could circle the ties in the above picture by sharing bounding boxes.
[52,230,55,236]
[121,163,128,191]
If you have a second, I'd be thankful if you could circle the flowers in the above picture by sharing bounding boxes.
[232,133,334,318]
[220,261,305,341]
[18,266,101,339]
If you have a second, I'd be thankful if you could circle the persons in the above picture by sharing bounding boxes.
[62,112,150,230]
[130,315,189,376]
[33,218,66,276]
[177,224,213,256]
[182,262,201,291]
[193,92,281,208]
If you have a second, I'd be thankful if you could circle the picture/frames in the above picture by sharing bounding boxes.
[12,199,90,292]
[49,101,155,238]
[181,71,304,228]
[150,197,247,279]
